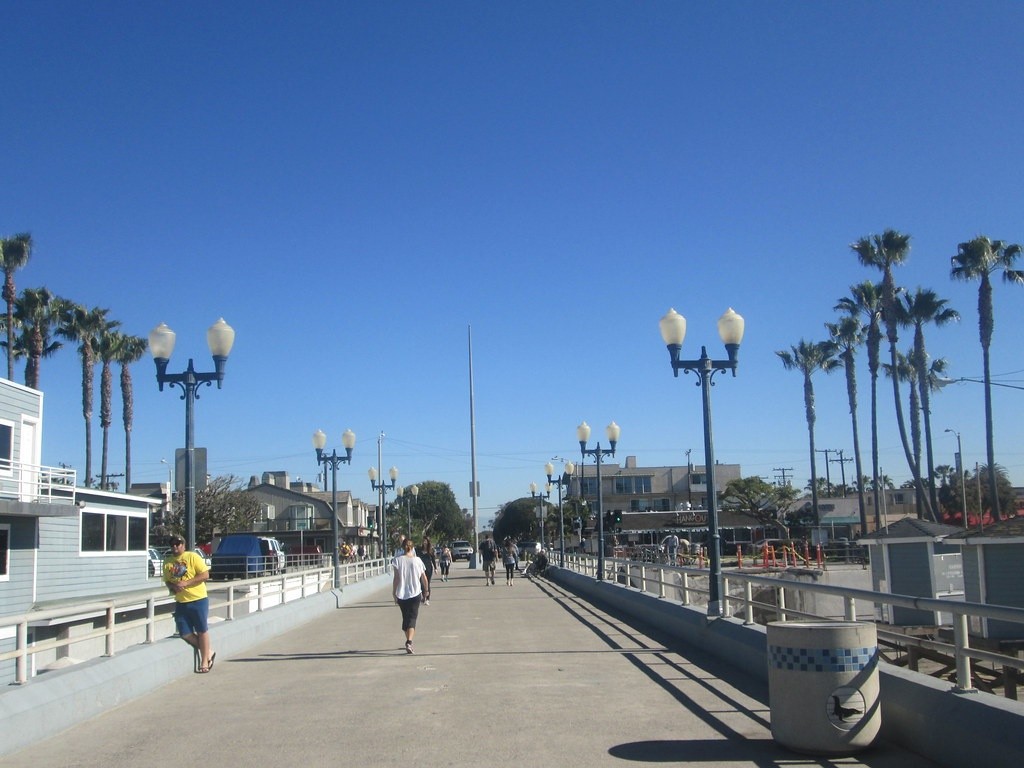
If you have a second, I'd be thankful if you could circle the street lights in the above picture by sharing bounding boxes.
[366,466,399,574]
[385,500,400,557]
[529,479,554,551]
[545,459,575,568]
[576,421,621,580]
[395,485,420,540]
[945,428,968,529]
[161,459,172,511]
[312,428,356,589]
[147,315,237,552]
[655,305,747,615]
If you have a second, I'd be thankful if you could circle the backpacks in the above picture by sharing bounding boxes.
[343,547,348,554]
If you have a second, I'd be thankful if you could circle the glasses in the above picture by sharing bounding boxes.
[170,541,181,548]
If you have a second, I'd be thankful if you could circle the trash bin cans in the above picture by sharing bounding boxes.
[766,620,882,756]
[321,555,332,568]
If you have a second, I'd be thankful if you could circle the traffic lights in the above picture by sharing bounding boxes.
[367,516,374,529]
[613,510,622,533]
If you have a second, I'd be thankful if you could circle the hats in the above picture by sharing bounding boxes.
[169,536,185,546]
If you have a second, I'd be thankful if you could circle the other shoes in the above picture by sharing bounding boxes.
[511,581,513,586]
[406,645,413,654]
[492,581,495,584]
[486,583,489,586]
[507,581,509,585]
[446,579,448,581]
[423,600,429,606]
[441,578,444,582]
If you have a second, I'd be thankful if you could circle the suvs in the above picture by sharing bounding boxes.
[448,540,474,562]
[257,536,286,576]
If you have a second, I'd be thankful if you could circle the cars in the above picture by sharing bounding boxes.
[147,546,207,560]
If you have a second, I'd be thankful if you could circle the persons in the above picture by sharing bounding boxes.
[391,539,429,653]
[668,533,679,566]
[478,535,498,586]
[579,538,587,554]
[502,539,519,586]
[395,536,438,606]
[357,545,364,560]
[439,545,452,583]
[162,534,216,673]
[521,542,547,577]
[339,541,356,564]
[315,545,322,553]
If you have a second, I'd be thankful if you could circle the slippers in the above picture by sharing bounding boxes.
[199,667,209,674]
[209,652,216,669]
